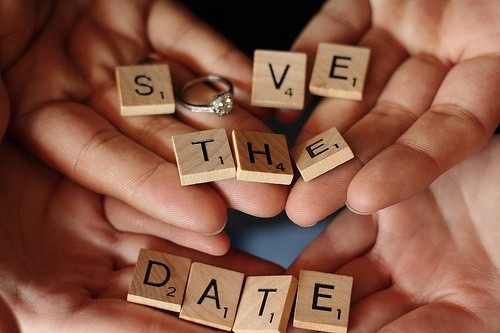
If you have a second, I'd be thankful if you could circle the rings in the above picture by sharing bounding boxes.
[178,74,235,117]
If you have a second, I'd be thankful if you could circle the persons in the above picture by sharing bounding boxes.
[0,124,500,333]
[1,0,500,236]
